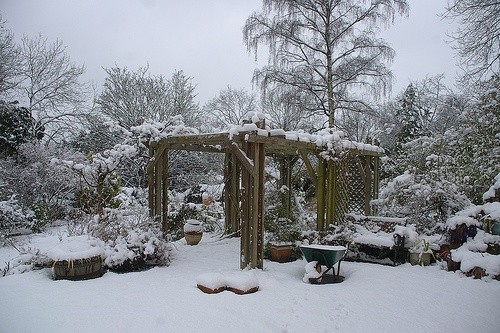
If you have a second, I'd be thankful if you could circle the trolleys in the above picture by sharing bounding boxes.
[291,240,351,284]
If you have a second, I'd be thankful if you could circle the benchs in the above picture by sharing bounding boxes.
[331,214,407,267]
